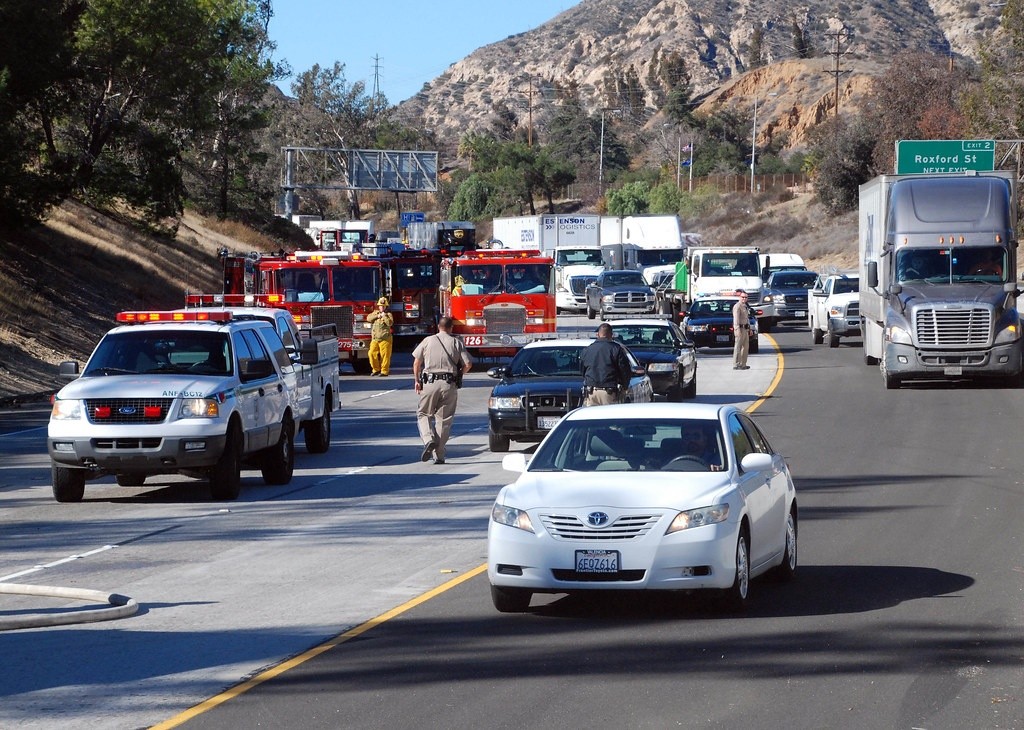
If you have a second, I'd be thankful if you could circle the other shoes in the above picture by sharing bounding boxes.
[378,373,388,376]
[421,440,437,462]
[370,371,379,376]
[733,366,750,370]
[433,458,445,464]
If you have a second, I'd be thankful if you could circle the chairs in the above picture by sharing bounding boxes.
[700,304,711,312]
[536,358,559,373]
[589,429,626,473]
[652,330,667,344]
[710,267,724,274]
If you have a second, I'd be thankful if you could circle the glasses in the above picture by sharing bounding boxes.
[742,297,748,298]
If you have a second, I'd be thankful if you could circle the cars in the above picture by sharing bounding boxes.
[677,293,763,353]
[585,270,659,320]
[602,312,699,403]
[764,270,822,327]
[486,338,654,454]
[487,401,800,612]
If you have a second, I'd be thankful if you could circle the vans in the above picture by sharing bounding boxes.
[758,253,808,286]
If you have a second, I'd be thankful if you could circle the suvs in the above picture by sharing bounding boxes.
[47,310,299,503]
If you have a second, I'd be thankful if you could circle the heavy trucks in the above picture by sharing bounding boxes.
[648,245,776,332]
[857,169,1024,392]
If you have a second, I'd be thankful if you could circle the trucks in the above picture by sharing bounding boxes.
[492,213,687,316]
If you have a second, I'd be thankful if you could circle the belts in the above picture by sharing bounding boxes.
[433,374,443,379]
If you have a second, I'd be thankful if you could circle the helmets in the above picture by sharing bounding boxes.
[377,296,390,307]
[454,274,465,286]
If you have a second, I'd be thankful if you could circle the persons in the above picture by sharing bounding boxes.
[581,323,631,406]
[411,317,472,464]
[367,297,393,377]
[681,424,721,466]
[733,292,753,369]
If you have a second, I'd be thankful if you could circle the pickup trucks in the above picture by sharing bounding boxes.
[806,272,862,348]
[168,306,342,457]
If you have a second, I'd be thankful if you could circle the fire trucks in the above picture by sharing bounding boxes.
[215,248,387,376]
[311,220,557,358]
